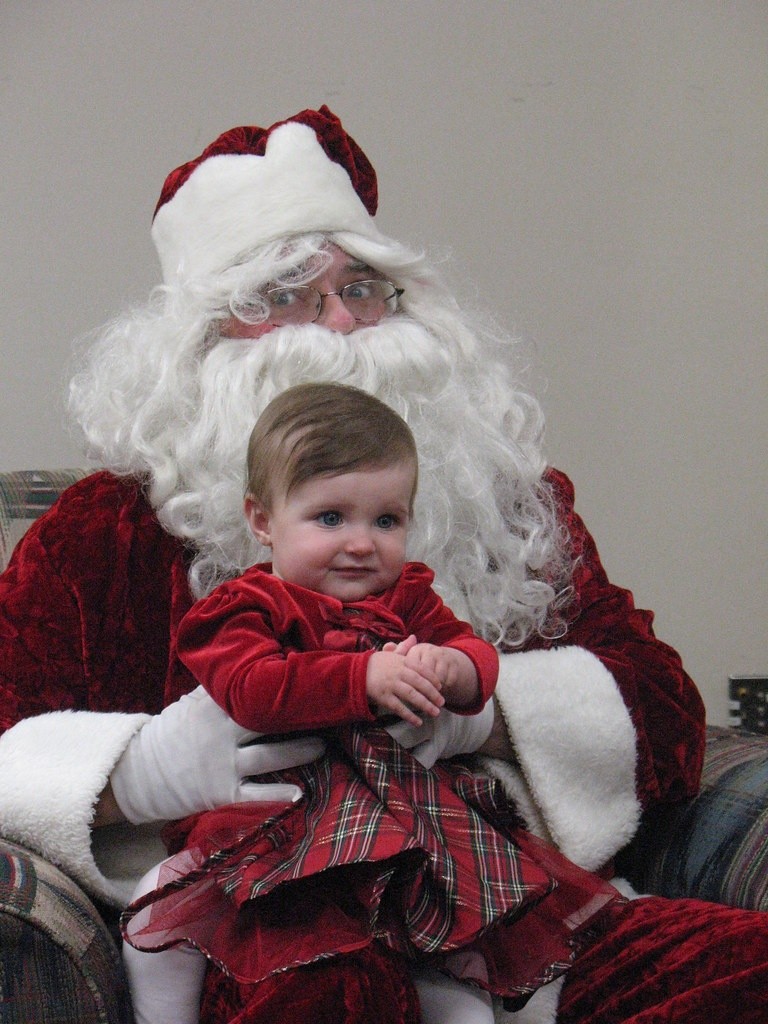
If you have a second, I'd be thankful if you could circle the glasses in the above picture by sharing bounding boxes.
[259,279,405,327]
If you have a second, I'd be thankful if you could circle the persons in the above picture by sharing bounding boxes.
[120,382,623,1023]
[0,105,768,1024]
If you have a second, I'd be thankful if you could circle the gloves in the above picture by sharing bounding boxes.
[109,685,325,827]
[377,697,495,770]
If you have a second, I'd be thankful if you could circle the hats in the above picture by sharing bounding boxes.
[150,105,377,293]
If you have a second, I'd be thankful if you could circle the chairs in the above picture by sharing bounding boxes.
[2,470,767,1023]
